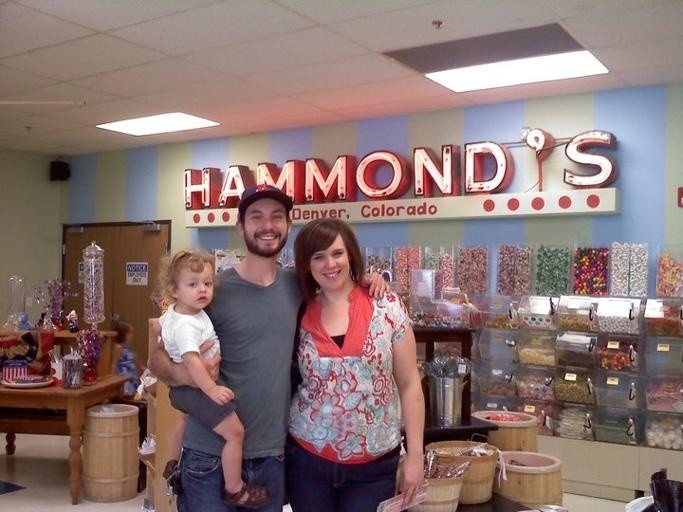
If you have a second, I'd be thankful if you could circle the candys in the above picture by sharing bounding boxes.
[84,252,107,324]
[394,241,683,372]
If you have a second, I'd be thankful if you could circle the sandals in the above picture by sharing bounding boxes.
[223,483,270,508]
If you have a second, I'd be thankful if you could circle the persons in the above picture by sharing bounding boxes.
[284,219,427,512]
[112,322,148,397]
[153,248,269,509]
[149,184,390,512]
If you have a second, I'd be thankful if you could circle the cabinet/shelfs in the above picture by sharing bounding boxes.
[405,319,530,511]
[143,318,186,512]
[0,326,131,507]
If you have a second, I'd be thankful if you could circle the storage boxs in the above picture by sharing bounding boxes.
[460,286,682,451]
[403,268,466,325]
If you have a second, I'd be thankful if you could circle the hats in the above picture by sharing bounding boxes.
[239,185,292,213]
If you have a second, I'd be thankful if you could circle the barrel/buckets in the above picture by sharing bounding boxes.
[410,411,563,512]
[81,405,141,504]
[421,362,464,427]
